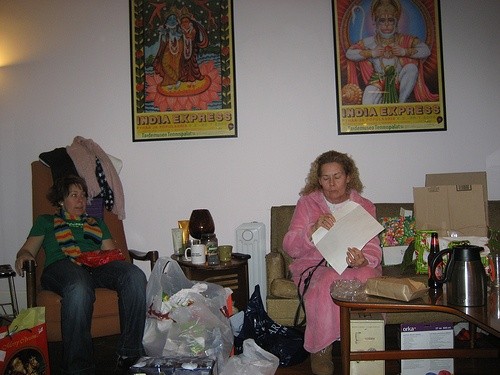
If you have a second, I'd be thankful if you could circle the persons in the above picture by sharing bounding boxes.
[14,175,147,375]
[282,151,383,375]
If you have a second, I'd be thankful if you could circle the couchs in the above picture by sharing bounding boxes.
[264,200,500,327]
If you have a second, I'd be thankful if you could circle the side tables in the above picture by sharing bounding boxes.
[171,252,249,313]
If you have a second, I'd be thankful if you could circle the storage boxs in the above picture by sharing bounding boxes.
[130,356,218,375]
[413,171,490,239]
[383,246,416,266]
[350,313,385,375]
[401,321,454,375]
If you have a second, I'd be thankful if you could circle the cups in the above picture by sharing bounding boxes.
[185,245,207,264]
[218,245,232,262]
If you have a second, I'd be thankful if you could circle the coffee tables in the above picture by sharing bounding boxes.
[330,276,500,375]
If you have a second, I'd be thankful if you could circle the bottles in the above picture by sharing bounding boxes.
[427,233,443,289]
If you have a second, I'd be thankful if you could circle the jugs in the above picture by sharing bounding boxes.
[430,244,488,307]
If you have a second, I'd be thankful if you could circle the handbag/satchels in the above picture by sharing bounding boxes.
[219,338,279,375]
[234,284,310,368]
[76,249,126,268]
[0,323,50,375]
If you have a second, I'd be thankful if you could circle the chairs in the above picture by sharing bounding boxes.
[23,161,158,342]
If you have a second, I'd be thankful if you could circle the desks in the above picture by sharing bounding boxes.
[0,264,19,319]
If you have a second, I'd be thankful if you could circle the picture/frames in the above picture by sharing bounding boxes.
[129,0,237,142]
[331,0,447,135]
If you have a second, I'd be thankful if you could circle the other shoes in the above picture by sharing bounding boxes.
[114,353,141,372]
